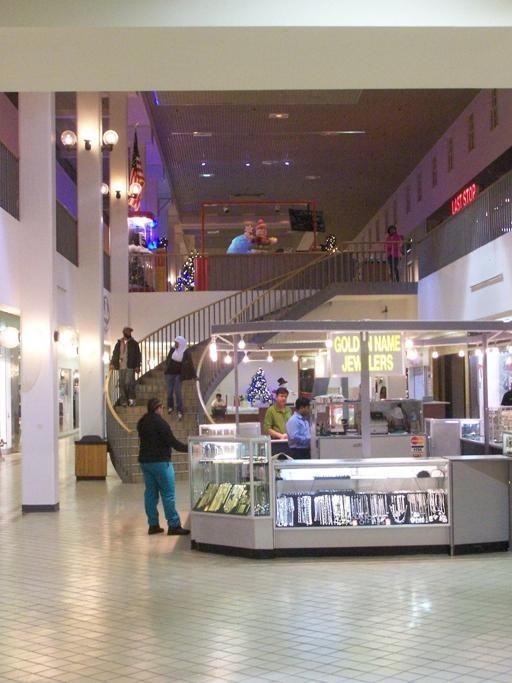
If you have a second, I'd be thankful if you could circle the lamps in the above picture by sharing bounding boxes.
[237,334,246,350]
[60,129,142,199]
[242,351,250,362]
[224,351,232,363]
[210,334,219,363]
[403,334,512,363]
[291,351,299,362]
[325,334,333,349]
[265,351,273,362]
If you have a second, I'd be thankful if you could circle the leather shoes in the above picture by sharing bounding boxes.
[148,525,164,534]
[168,526,190,535]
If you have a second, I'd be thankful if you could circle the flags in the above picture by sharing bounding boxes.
[127,128,146,211]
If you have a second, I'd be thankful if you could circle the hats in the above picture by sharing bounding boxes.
[123,327,133,331]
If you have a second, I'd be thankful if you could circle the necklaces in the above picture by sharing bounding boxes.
[275,489,447,524]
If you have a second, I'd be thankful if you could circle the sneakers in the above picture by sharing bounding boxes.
[177,410,183,419]
[168,407,174,414]
[129,399,135,407]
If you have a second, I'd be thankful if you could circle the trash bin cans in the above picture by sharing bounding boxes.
[74,435,108,481]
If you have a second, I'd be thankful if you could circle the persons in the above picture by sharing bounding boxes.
[162,335,189,420]
[211,393,226,416]
[108,326,142,406]
[500,382,512,406]
[263,389,292,438]
[135,396,189,536]
[285,398,311,457]
[383,224,404,282]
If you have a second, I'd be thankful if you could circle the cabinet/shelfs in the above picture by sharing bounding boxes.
[187,422,452,556]
